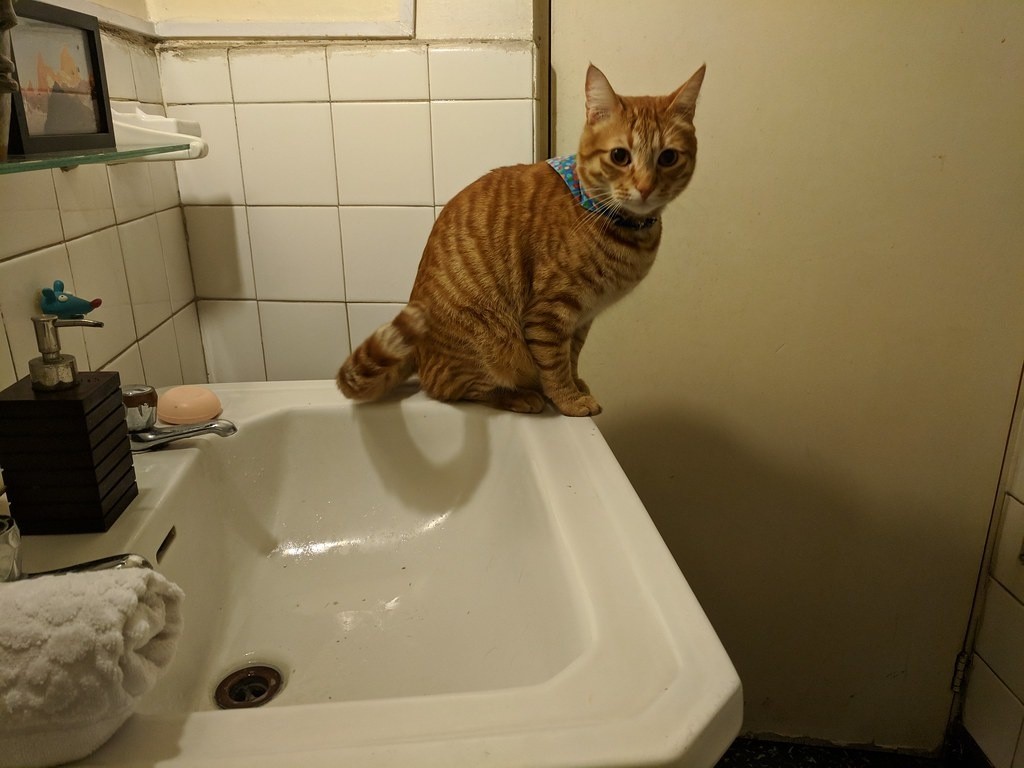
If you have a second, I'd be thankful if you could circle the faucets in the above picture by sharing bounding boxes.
[119,383,239,455]
[0,511,158,584]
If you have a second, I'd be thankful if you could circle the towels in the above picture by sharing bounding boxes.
[0,566,188,768]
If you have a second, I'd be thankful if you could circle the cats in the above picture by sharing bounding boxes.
[336,62,708,416]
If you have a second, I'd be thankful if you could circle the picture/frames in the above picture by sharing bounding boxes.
[9,0,116,157]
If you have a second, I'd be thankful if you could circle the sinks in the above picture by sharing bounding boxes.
[104,388,610,725]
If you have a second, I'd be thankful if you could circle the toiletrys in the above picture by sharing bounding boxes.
[0,312,140,538]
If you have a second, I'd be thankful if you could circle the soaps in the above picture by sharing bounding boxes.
[157,383,223,426]
